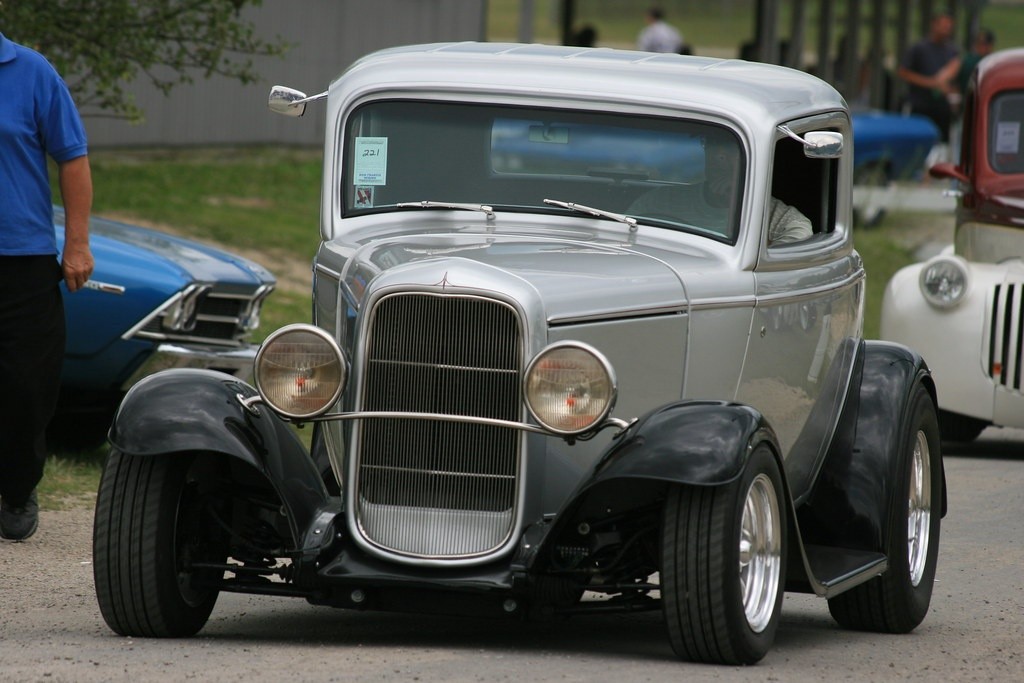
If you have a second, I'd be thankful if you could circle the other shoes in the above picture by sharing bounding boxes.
[0,482,38,543]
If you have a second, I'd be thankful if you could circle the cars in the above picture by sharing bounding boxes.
[880,47,1024,457]
[41,198,279,433]
[481,104,943,229]
[88,39,953,670]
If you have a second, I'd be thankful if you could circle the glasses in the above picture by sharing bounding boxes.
[700,135,740,151]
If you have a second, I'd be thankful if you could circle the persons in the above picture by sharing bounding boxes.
[565,6,994,183]
[616,120,816,253]
[0,33,93,541]
